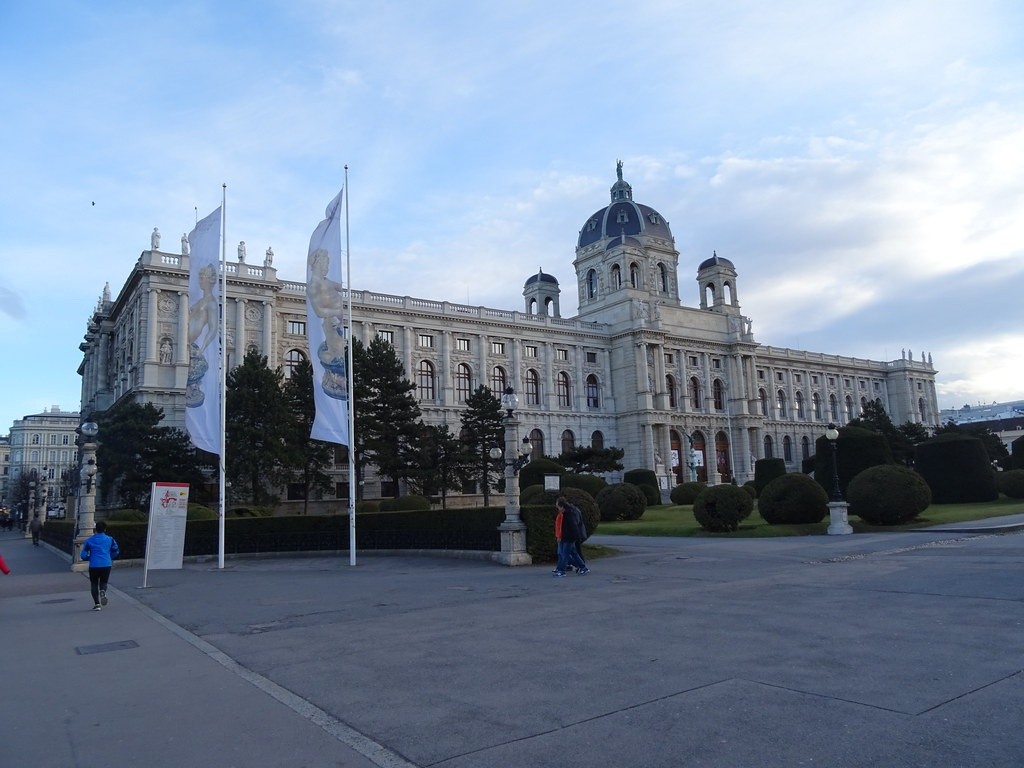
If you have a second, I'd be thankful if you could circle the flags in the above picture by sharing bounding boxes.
[186,202,226,456]
[304,187,350,447]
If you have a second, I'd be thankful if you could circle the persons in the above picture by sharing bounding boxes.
[161,341,172,363]
[746,317,753,334]
[29,512,43,546]
[151,227,161,251]
[265,247,274,266]
[552,497,590,578]
[639,299,649,319]
[654,300,661,319]
[0,515,29,533]
[85,281,110,327]
[238,241,246,261]
[164,297,173,310]
[181,233,189,255]
[80,521,119,610]
[901,348,932,362]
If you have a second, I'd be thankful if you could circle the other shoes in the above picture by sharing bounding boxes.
[100,590,107,606]
[552,570,566,577]
[567,565,573,571]
[92,604,101,611]
[576,568,589,576]
